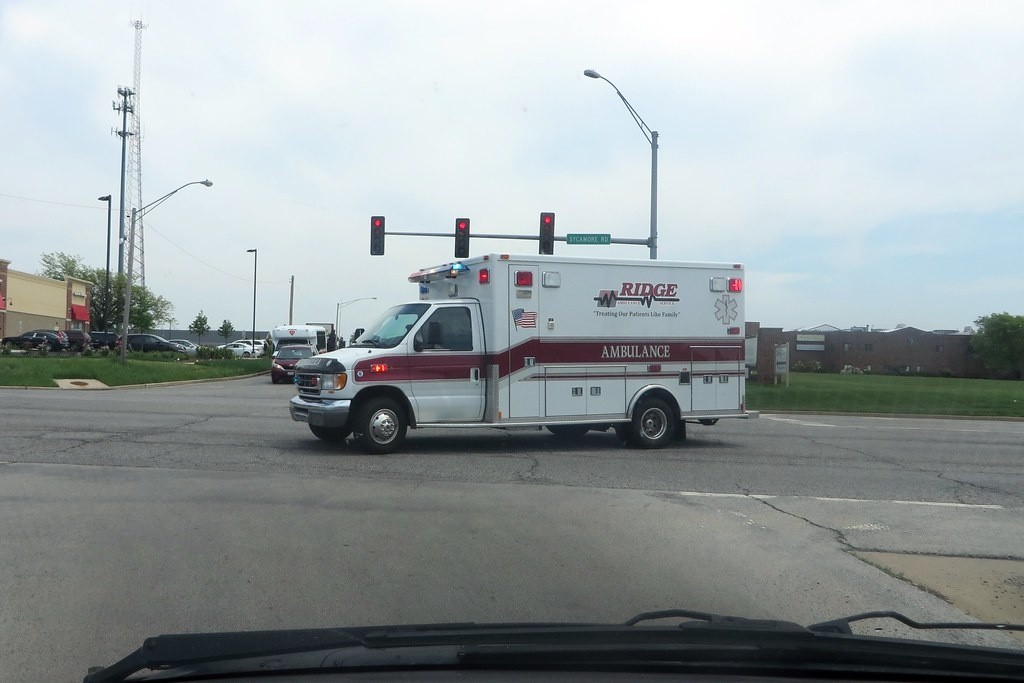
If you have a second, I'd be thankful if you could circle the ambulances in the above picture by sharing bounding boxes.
[288,251,746,456]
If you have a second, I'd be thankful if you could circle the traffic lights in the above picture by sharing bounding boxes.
[456,217,471,257]
[369,217,384,253]
[539,210,556,254]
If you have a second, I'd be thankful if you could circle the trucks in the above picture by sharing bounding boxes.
[272,326,326,357]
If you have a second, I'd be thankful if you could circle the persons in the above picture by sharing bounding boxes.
[420,316,469,351]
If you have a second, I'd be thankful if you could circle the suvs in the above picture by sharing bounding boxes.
[87,330,119,350]
[64,330,93,351]
[233,340,270,357]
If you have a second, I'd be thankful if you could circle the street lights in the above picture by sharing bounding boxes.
[583,69,658,260]
[247,249,257,358]
[99,195,112,354]
[120,181,214,359]
[334,297,378,350]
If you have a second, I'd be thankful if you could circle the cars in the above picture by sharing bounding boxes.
[30,329,70,352]
[2,333,63,352]
[116,334,187,354]
[168,339,198,357]
[272,345,318,385]
[214,343,261,359]
